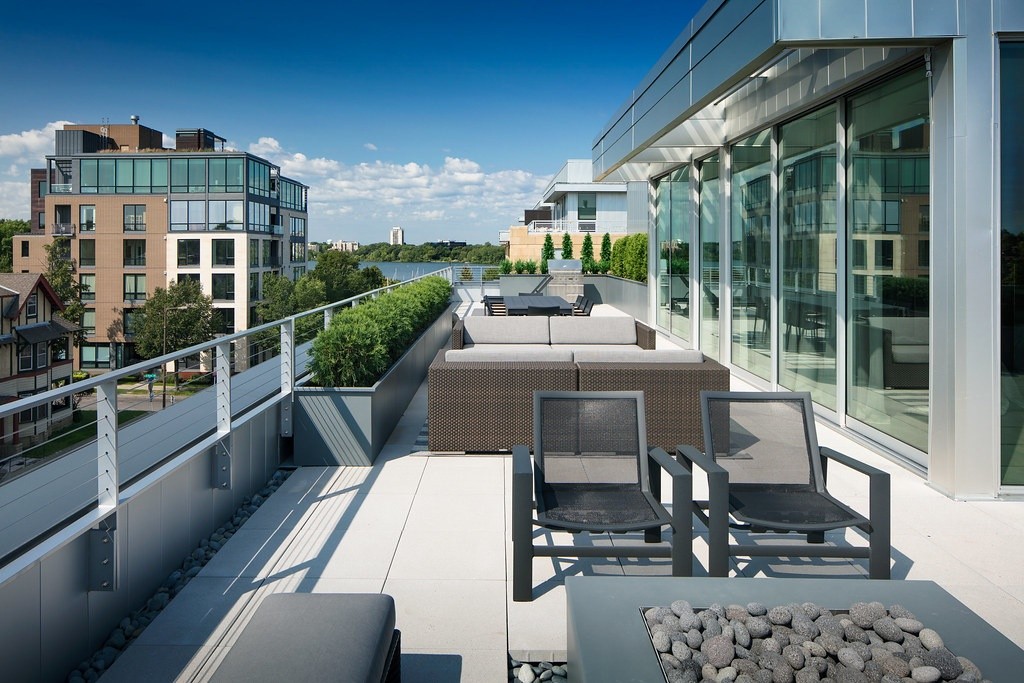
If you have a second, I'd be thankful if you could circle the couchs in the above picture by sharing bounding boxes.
[550,316,657,350]
[427,347,578,457]
[452,316,552,350]
[574,347,731,456]
[853,316,929,390]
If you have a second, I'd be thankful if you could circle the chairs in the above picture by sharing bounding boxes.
[857,296,877,321]
[513,389,693,604]
[484,293,594,317]
[753,295,827,354]
[703,282,719,303]
[679,276,689,298]
[676,390,892,580]
[871,306,900,317]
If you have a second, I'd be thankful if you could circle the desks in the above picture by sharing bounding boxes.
[785,296,908,351]
[503,296,574,316]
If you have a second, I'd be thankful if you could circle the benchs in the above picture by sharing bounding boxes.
[205,590,402,683]
